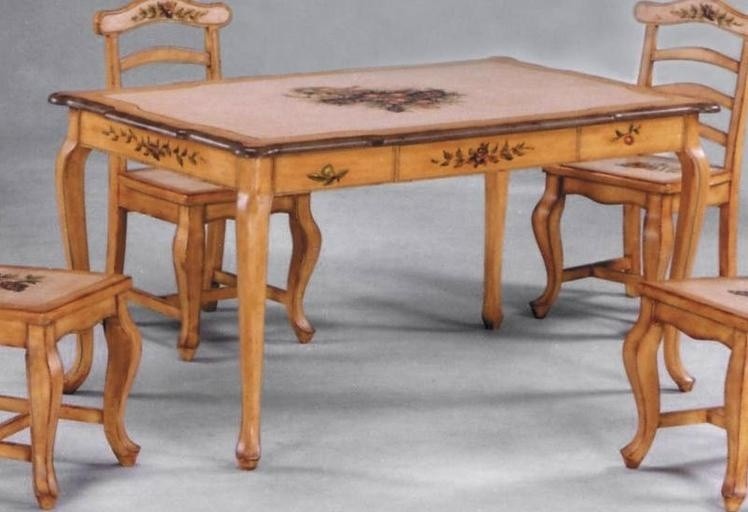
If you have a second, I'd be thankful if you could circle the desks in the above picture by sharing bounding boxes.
[46,54,721,470]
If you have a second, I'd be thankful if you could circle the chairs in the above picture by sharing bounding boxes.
[619,276,748,511]
[2,264,144,511]
[528,4,748,319]
[93,3,323,361]
